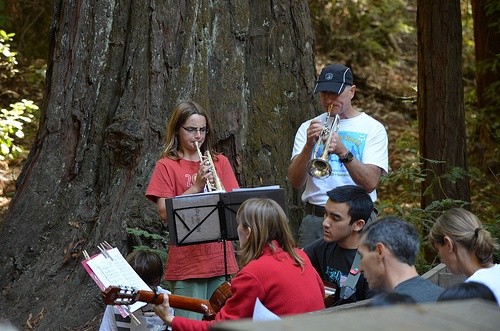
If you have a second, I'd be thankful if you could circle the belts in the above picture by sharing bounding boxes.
[305,201,325,217]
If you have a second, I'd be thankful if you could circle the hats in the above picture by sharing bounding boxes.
[312,64,354,95]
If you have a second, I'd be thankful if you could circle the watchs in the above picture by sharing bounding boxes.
[339,150,353,163]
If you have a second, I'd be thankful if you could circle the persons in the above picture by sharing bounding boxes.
[300,185,374,307]
[152,197,325,331]
[288,64,388,308]
[357,215,444,307]
[98,250,174,331]
[428,207,500,308]
[145,100,240,320]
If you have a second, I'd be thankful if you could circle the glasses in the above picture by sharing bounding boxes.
[180,125,209,133]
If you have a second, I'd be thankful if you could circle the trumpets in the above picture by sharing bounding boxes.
[194,140,226,192]
[306,104,341,179]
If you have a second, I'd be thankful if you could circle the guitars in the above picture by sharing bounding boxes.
[101,282,231,322]
